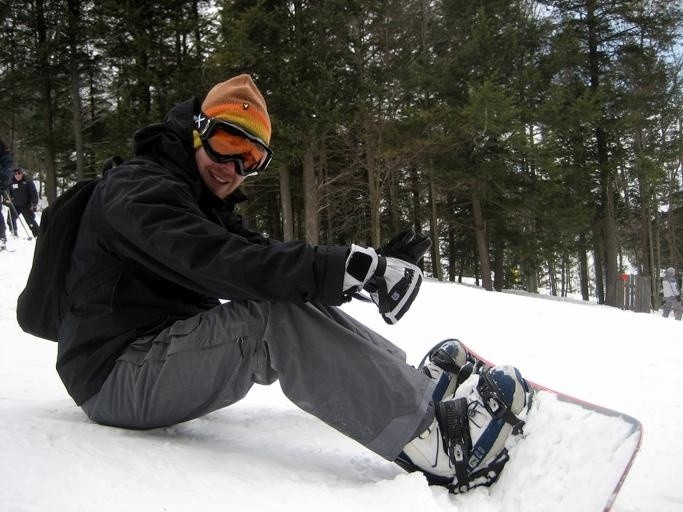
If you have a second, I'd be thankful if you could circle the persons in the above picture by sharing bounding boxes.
[662,267,682,320]
[0,140,12,249]
[8,167,40,237]
[56,74,526,489]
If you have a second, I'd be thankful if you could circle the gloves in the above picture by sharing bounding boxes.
[30,203,36,212]
[676,296,680,301]
[344,230,432,324]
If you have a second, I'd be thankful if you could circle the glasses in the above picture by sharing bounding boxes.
[192,110,273,176]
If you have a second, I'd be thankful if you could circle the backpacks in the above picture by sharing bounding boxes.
[17,155,124,343]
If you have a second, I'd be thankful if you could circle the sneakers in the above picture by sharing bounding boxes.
[405,365,527,478]
[418,339,467,400]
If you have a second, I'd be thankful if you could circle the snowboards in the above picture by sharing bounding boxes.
[10,233,38,240]
[416,338,644,512]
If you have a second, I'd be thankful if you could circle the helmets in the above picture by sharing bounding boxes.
[667,267,676,273]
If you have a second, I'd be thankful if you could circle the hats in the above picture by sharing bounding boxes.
[192,74,271,149]
